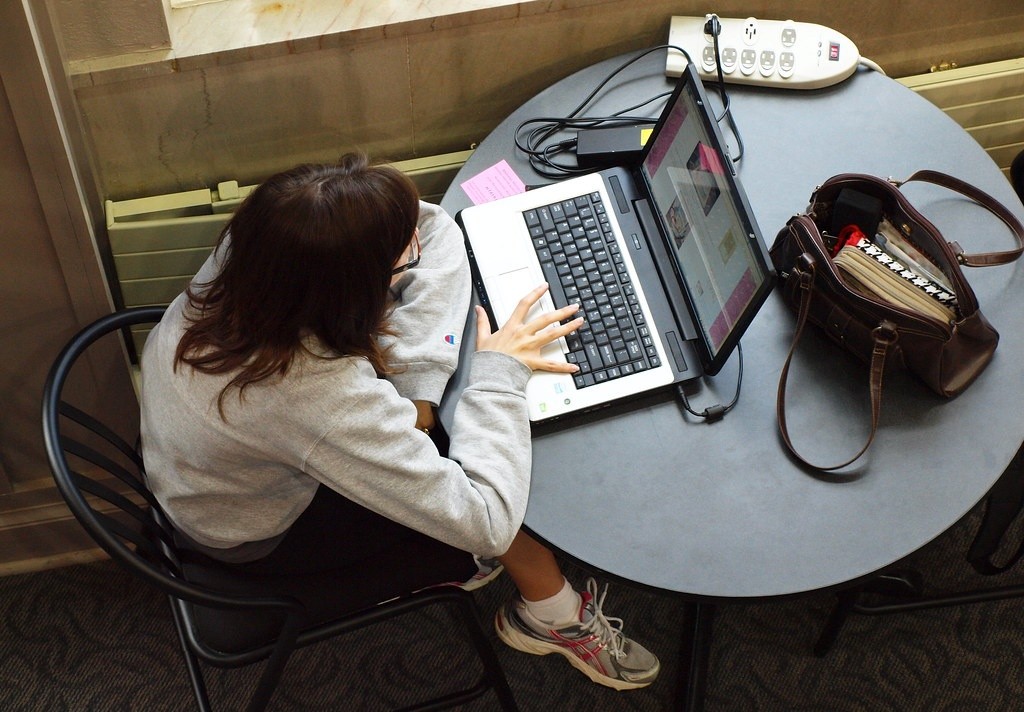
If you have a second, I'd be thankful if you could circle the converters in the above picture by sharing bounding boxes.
[576,125,656,168]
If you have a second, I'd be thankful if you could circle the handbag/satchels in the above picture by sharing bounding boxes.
[766,169,1024,471]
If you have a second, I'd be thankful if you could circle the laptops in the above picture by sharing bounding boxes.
[459,64,776,425]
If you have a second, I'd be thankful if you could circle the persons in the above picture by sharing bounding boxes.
[140,151,662,690]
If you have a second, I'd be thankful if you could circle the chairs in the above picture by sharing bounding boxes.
[42,306,516,712]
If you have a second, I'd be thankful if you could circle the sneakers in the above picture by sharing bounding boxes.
[495,576,660,692]
[377,552,504,606]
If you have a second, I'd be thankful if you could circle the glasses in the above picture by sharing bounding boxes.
[392,230,421,276]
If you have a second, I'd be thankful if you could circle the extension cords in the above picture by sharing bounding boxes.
[665,15,860,89]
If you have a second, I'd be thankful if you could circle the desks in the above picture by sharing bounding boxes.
[438,45,1024,712]
